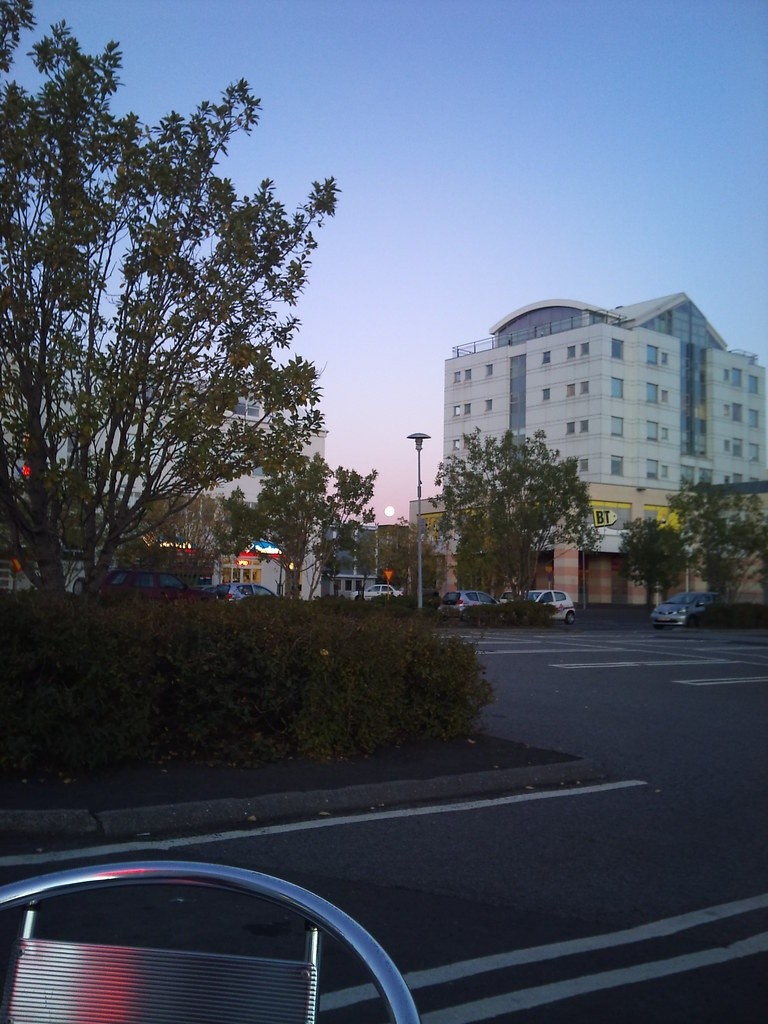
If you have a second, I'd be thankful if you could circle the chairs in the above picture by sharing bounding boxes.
[0,858,420,1024]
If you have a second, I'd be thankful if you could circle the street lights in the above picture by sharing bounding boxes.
[407,432,431,608]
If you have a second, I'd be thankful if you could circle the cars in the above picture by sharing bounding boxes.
[438,590,501,621]
[194,586,215,593]
[96,569,215,603]
[350,584,403,602]
[215,583,276,601]
[498,591,513,602]
[518,591,575,624]
[651,591,721,628]
